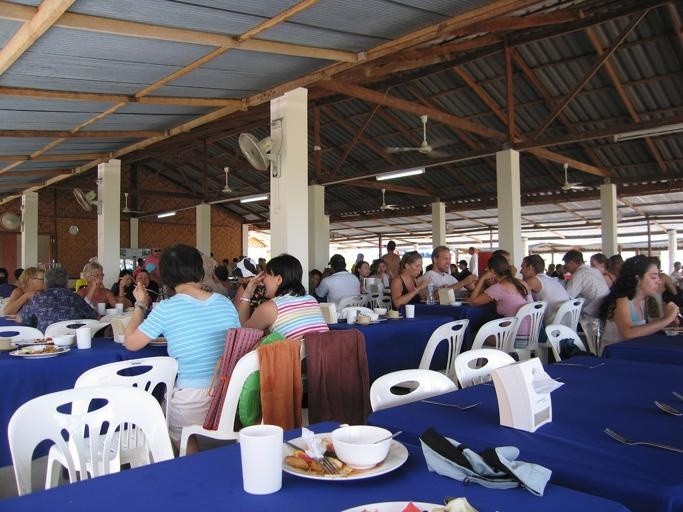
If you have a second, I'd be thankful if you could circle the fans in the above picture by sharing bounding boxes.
[120,193,146,213]
[199,167,251,193]
[73,187,102,212]
[1,211,24,233]
[238,133,281,178]
[384,115,454,158]
[560,162,588,191]
[379,189,399,209]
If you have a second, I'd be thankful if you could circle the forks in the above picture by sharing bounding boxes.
[654,399,683,417]
[605,427,683,454]
[551,361,605,370]
[135,285,167,296]
[285,438,340,475]
[421,398,481,410]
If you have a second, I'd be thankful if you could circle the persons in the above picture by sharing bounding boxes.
[1,241,330,456]
[308,240,683,366]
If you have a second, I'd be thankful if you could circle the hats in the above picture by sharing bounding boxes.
[328,254,345,265]
[457,260,467,266]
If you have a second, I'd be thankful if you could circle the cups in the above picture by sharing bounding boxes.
[347,309,357,324]
[76,328,91,349]
[97,302,105,315]
[0,338,11,349]
[406,305,414,319]
[239,425,284,494]
[363,278,375,293]
[115,303,123,314]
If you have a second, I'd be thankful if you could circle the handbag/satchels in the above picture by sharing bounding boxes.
[204,327,264,430]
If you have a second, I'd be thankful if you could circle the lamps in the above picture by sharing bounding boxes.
[614,124,683,143]
[376,167,425,181]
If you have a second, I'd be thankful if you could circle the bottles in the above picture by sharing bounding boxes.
[426,278,435,305]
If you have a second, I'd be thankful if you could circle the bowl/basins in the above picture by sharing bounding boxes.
[358,308,399,322]
[53,334,76,346]
[333,425,391,469]
[107,309,118,316]
[450,301,462,306]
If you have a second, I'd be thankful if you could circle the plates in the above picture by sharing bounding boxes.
[149,342,167,347]
[15,337,53,346]
[341,501,445,512]
[284,432,408,480]
[357,322,380,325]
[9,345,70,359]
